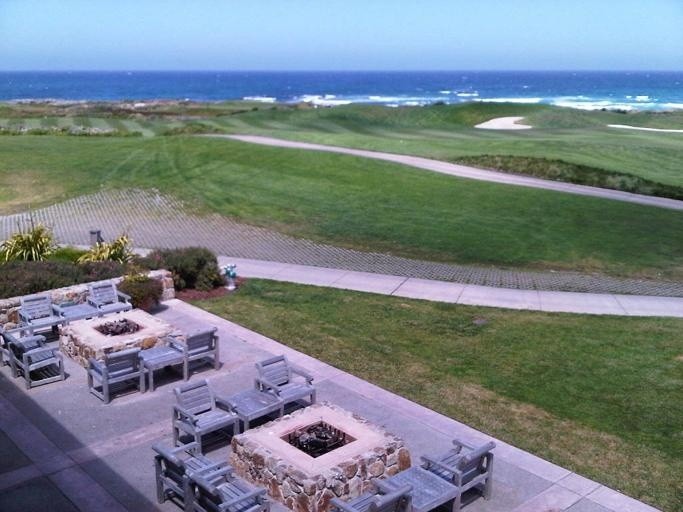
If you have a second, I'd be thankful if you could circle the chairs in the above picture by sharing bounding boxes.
[0,281,219,406]
